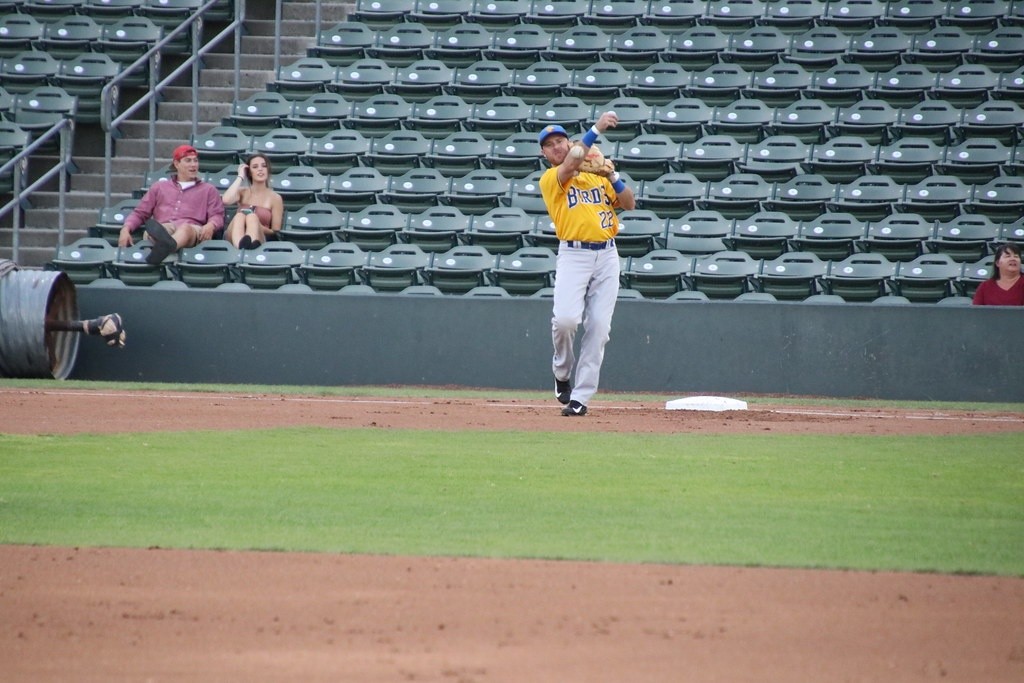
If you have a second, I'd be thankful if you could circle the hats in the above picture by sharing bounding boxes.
[537,124,570,146]
[168,145,198,169]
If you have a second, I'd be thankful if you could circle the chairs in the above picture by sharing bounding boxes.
[1,0,1024,307]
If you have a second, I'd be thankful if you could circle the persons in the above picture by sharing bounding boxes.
[118,145,225,266]
[222,154,283,250]
[538,112,635,416]
[972,242,1024,306]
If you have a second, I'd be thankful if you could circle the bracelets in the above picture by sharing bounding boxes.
[238,176,244,181]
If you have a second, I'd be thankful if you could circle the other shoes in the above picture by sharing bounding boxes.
[145,240,174,264]
[251,240,260,250]
[238,234,252,249]
[144,218,178,251]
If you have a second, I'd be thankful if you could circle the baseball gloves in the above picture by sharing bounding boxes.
[575,143,615,178]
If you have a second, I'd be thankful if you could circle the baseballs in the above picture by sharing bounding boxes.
[571,144,584,158]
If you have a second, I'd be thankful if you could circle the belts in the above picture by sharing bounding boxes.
[567,240,614,250]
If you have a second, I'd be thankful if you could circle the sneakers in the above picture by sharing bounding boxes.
[561,400,590,416]
[554,375,572,404]
